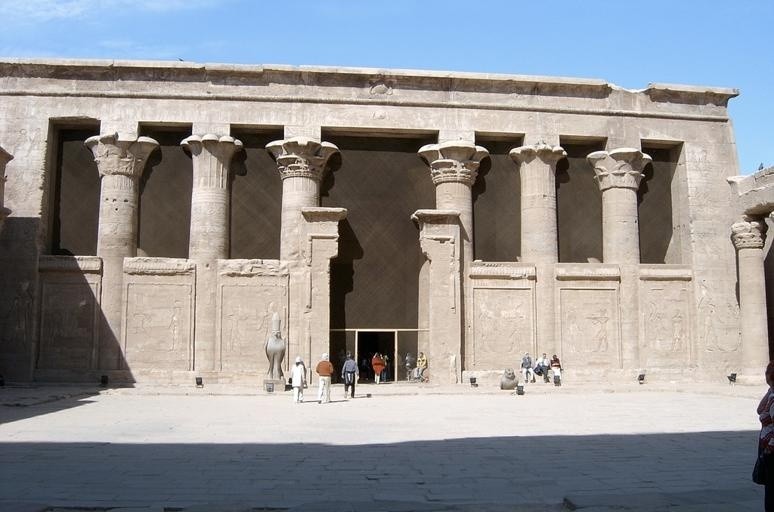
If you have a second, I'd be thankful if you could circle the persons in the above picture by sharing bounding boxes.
[534,352,551,384]
[753,360,774,511]
[551,355,563,384]
[330,347,429,398]
[518,352,534,383]
[291,356,308,404]
[315,353,334,405]
